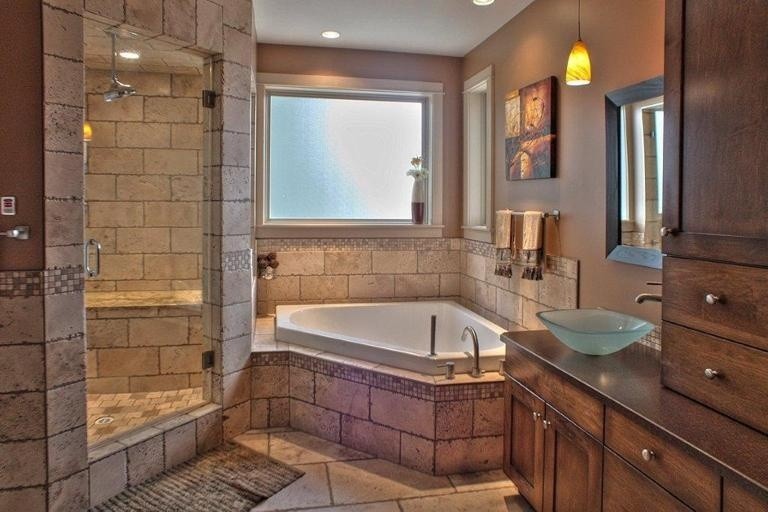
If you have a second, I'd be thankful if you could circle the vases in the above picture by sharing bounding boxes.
[411,179,425,224]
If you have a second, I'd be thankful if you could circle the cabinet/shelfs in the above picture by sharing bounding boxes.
[605,404,720,510]
[658,0,768,433]
[604,449,696,511]
[500,375,602,510]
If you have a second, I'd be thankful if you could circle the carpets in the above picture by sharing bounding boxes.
[85,438,305,512]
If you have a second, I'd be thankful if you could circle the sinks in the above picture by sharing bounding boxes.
[535,309,657,356]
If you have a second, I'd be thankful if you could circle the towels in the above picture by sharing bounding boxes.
[520,211,544,280]
[494,209,516,278]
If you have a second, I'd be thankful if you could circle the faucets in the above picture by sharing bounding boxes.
[460,326,482,377]
[634,281,663,305]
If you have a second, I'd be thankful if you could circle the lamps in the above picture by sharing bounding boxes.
[565,0,591,86]
[83,118,93,143]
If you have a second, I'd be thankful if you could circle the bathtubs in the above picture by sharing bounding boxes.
[274,299,509,375]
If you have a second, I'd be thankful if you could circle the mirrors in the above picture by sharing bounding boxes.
[604,75,666,269]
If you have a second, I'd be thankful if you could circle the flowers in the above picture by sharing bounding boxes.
[406,156,429,180]
